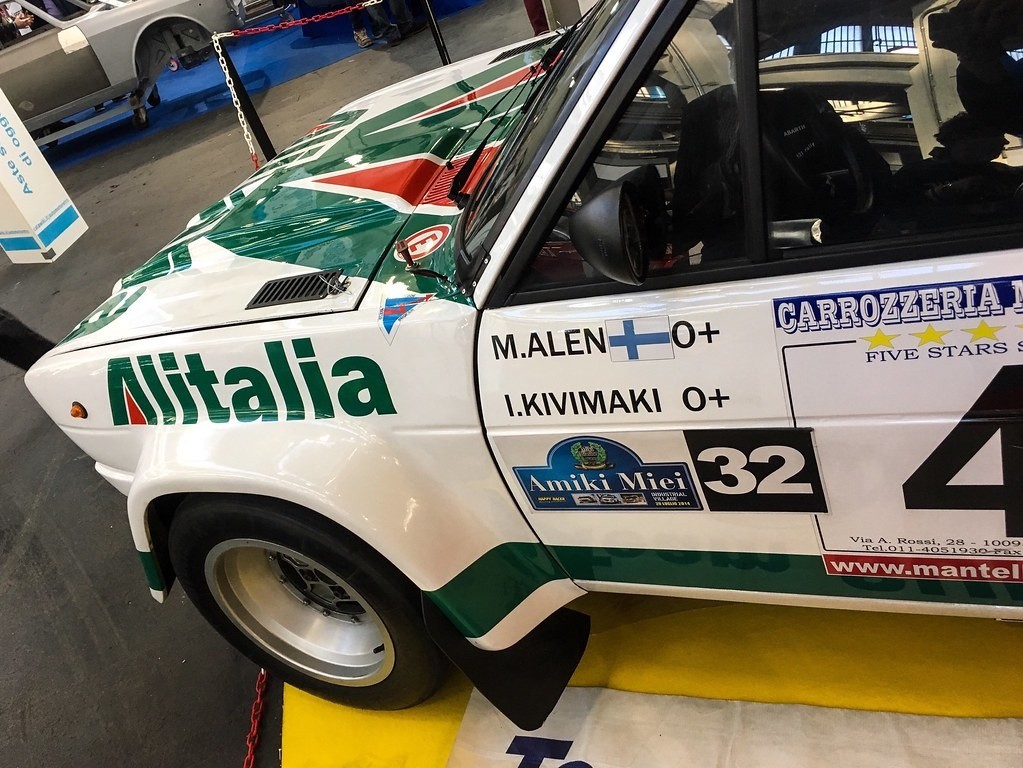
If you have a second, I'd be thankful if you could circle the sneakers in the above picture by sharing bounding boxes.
[371,23,399,39]
[354,27,372,48]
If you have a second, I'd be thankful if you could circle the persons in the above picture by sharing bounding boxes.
[346,0,430,48]
[0,3,76,147]
[22,0,130,113]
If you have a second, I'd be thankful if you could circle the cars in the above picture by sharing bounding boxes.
[24,0,1022,712]
[0,0,247,134]
